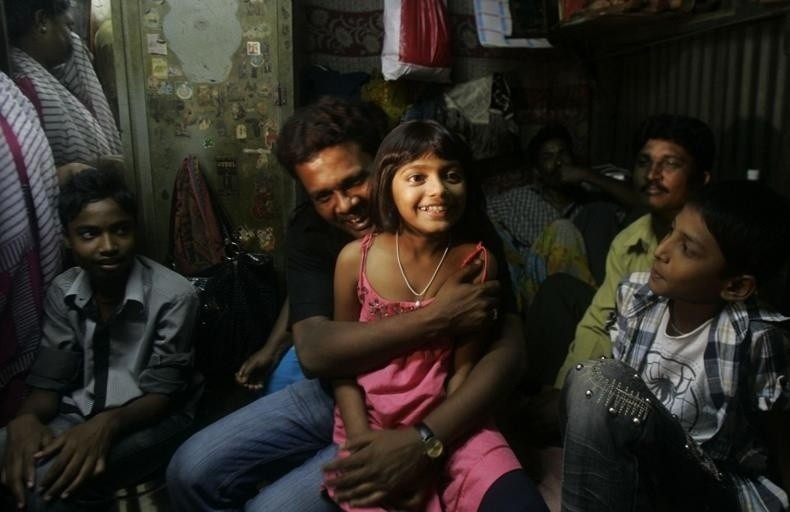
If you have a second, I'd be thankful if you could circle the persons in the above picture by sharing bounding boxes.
[2,1,126,219]
[168,97,550,512]
[551,114,724,446]
[555,182,789,512]
[484,118,652,452]
[1,164,198,512]
[1,74,65,425]
